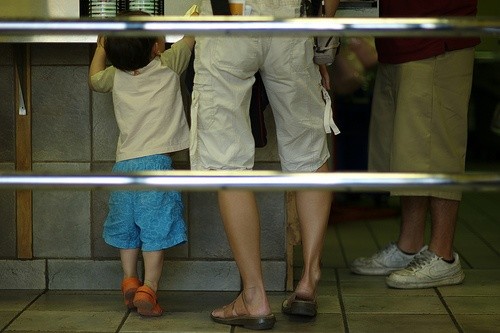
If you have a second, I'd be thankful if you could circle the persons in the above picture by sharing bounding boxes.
[189,0,340,330]
[351,0,480,289]
[87,9,200,317]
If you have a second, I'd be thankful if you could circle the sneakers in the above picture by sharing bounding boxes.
[133,286,163,318]
[350,244,428,275]
[121,276,141,308]
[386,248,462,289]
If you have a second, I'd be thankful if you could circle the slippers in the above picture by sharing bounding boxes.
[210,292,275,330]
[281,289,319,319]
